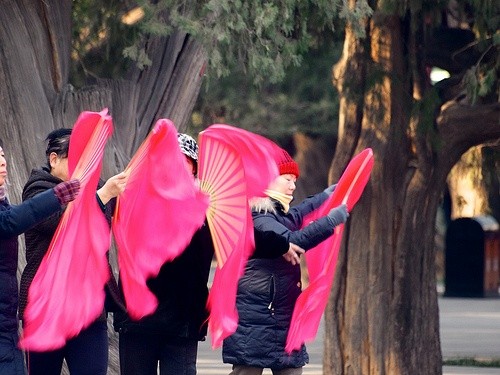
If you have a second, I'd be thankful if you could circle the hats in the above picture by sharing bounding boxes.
[278,148,300,180]
[177,132,199,160]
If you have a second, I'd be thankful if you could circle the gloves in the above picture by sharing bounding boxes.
[323,183,338,196]
[53,178,80,205]
[327,204,349,226]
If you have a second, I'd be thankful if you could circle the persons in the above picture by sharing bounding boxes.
[18,128,129,375]
[0,139,81,375]
[222,149,350,375]
[113,132,214,375]
